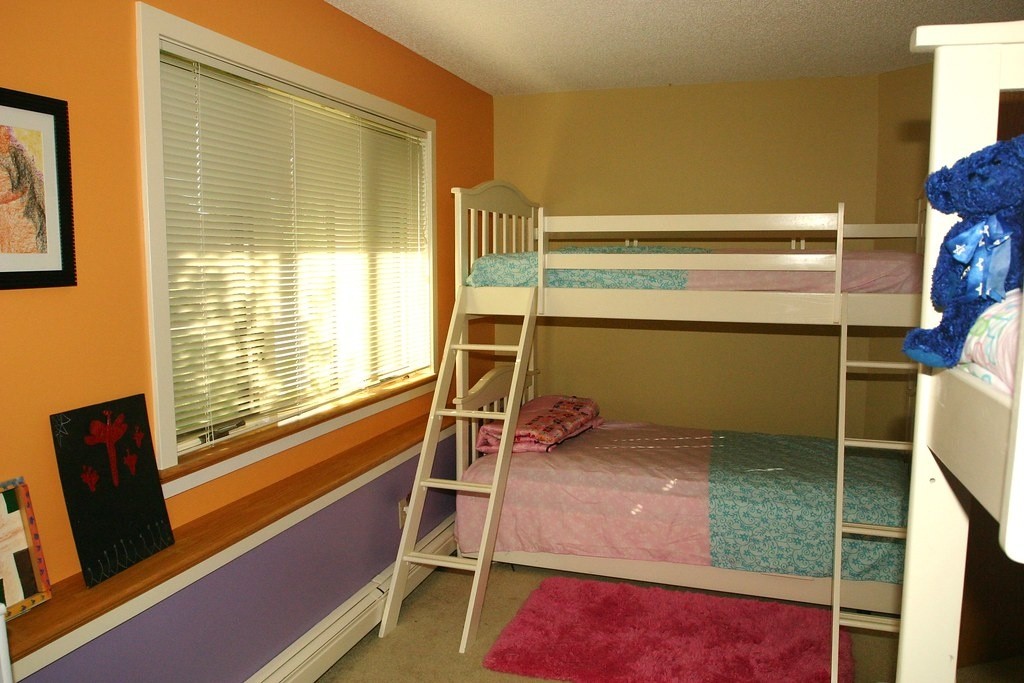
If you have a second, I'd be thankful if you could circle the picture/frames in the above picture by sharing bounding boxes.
[0,475,53,622]
[0,87,77,290]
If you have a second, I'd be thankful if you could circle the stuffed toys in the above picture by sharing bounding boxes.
[902,134,1024,369]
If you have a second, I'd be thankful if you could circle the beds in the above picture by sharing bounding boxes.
[451,19,1023,683]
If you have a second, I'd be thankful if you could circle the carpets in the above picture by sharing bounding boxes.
[482,578,856,683]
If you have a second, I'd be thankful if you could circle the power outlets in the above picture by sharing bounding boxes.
[398,497,409,530]
